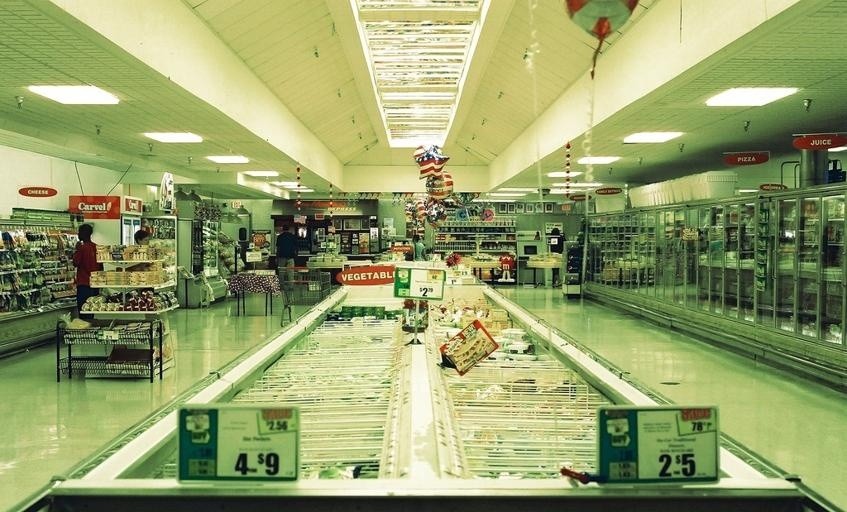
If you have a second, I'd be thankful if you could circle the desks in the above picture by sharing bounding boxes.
[526,262,564,289]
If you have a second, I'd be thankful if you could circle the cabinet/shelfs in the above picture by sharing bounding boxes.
[582,181,847,393]
[429,226,519,284]
[67,195,142,248]
[562,240,582,299]
[177,200,249,307]
[270,198,379,255]
[0,216,95,355]
[142,215,178,291]
[10,262,846,512]
[56,258,185,385]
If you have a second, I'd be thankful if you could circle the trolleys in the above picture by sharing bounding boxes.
[277,267,332,328]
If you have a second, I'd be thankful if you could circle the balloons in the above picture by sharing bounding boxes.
[404,144,453,230]
[563,0,640,45]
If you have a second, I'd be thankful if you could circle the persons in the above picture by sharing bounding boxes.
[548,228,567,289]
[412,234,428,261]
[72,223,103,320]
[276,225,299,291]
[133,230,150,247]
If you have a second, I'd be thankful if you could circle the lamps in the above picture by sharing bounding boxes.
[234,204,251,217]
